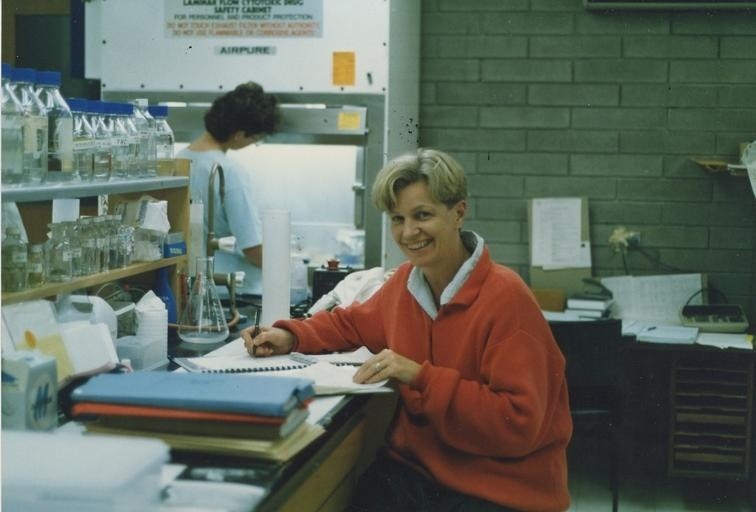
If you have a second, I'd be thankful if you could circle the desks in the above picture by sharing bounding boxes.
[38,353,401,512]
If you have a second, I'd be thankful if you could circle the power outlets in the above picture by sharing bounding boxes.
[614,232,640,252]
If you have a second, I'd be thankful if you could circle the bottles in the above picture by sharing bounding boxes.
[153,268,177,337]
[0,64,73,186]
[1,213,136,291]
[67,95,176,184]
[176,256,231,345]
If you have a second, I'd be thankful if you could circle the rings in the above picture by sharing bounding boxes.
[375,360,381,370]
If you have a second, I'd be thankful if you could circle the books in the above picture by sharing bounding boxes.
[0,428,184,512]
[565,294,754,350]
[72,371,328,464]
[168,346,394,397]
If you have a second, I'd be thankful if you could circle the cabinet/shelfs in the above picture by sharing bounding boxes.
[1,149,192,376]
[664,346,756,483]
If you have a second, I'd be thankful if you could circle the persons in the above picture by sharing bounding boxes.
[240,148,573,512]
[173,80,279,288]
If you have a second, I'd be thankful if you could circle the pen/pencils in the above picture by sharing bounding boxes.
[253,309,261,355]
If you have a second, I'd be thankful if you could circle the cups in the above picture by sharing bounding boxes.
[138,308,168,338]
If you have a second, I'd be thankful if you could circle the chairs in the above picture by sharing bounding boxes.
[542,317,632,512]
